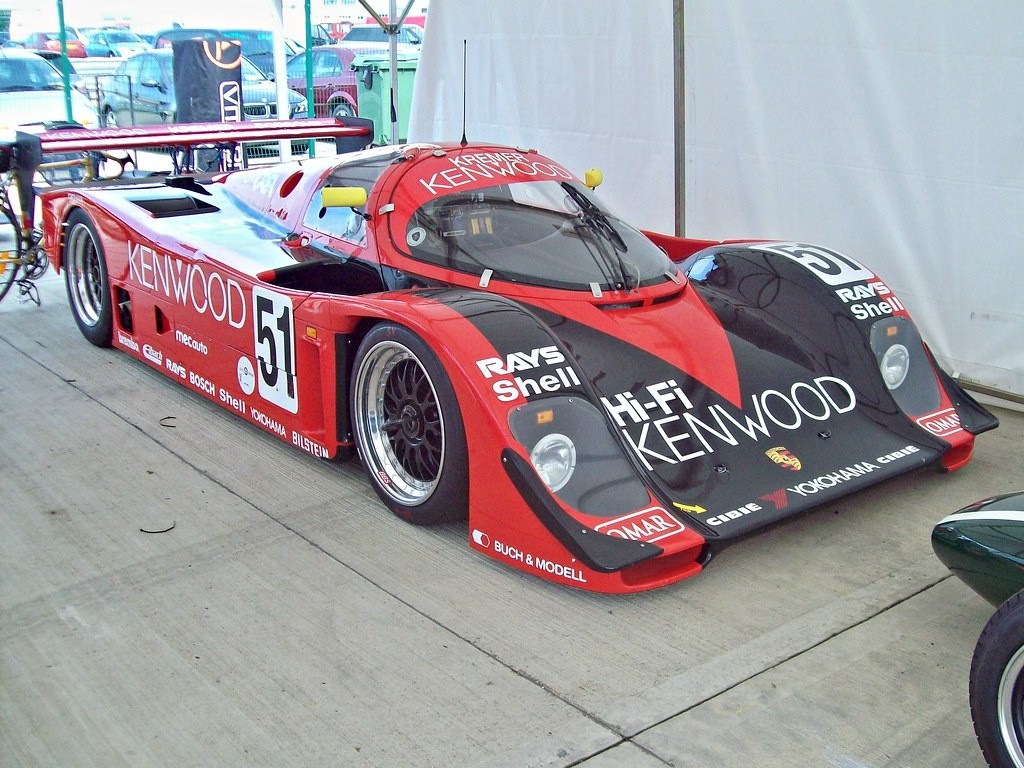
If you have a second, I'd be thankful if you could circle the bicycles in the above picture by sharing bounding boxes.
[0,141,138,304]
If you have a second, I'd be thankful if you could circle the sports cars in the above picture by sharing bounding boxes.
[15,119,1004,594]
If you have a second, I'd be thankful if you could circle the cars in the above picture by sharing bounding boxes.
[336,23,425,63]
[24,30,88,61]
[100,46,311,157]
[81,28,154,60]
[67,24,94,45]
[278,45,396,119]
[30,46,91,101]
[1,49,101,138]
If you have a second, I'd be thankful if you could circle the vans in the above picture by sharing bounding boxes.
[150,28,298,78]
